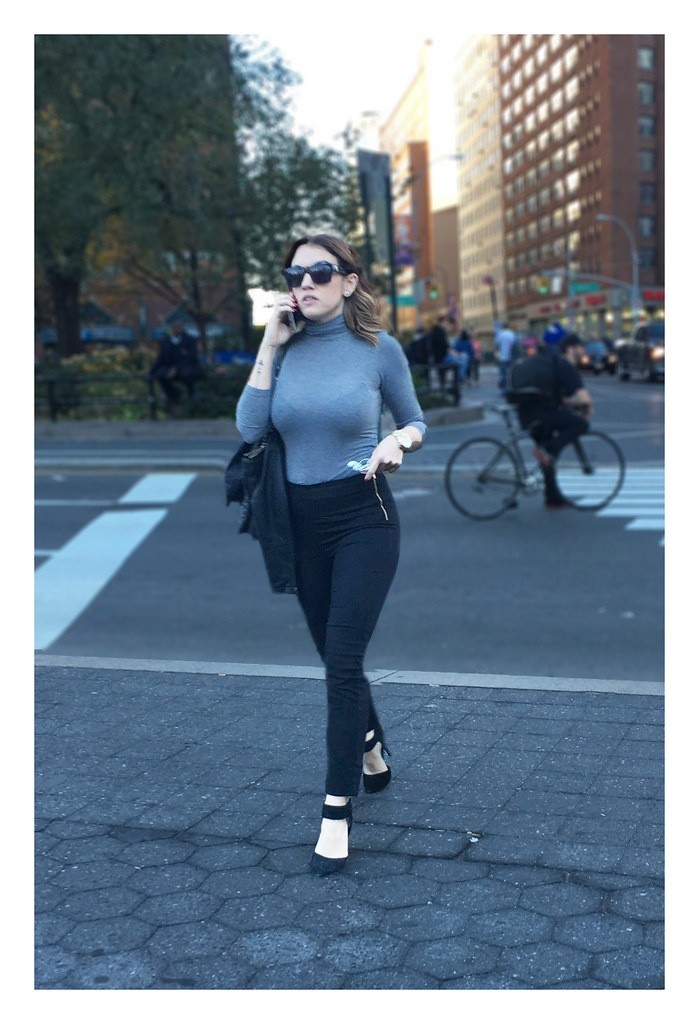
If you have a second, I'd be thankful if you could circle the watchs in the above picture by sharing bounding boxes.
[391,428,413,454]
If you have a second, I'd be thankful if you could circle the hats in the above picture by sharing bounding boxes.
[542,322,567,346]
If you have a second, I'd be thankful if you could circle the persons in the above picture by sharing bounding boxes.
[494,323,516,389]
[151,325,204,402]
[515,322,594,508]
[234,227,430,874]
[406,314,483,388]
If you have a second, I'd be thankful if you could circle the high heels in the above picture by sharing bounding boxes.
[311,798,353,877]
[362,721,392,794]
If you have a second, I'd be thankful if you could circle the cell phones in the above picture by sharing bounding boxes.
[288,301,301,332]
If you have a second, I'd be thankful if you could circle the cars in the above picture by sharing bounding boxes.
[570,320,665,381]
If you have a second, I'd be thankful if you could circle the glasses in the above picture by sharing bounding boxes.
[282,260,349,287]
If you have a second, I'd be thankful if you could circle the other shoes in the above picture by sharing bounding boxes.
[533,446,553,466]
[544,496,573,510]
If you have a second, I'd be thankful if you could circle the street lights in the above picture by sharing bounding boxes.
[597,214,639,323]
[364,111,421,332]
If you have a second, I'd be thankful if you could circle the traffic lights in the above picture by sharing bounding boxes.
[428,283,440,299]
[537,275,549,295]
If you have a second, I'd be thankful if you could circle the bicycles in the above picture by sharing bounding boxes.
[444,401,627,521]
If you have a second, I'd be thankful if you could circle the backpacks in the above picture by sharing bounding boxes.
[499,355,566,430]
